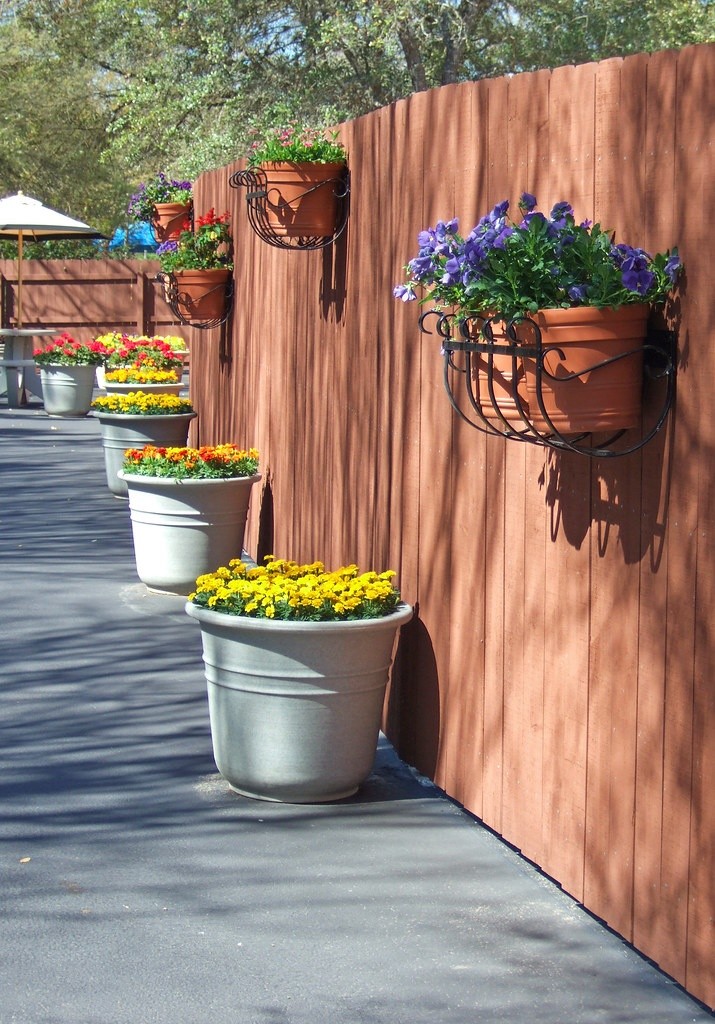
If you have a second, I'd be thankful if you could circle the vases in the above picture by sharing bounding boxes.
[149,201,191,243]
[251,160,345,238]
[35,360,187,398]
[117,470,262,595]
[88,409,198,500]
[184,599,414,804]
[166,268,229,320]
[466,305,650,436]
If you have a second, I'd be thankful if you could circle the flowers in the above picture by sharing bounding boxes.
[187,551,402,622]
[155,206,234,284]
[391,194,685,356]
[121,442,261,483]
[128,171,196,227]
[244,116,348,179]
[33,333,191,385]
[88,392,194,416]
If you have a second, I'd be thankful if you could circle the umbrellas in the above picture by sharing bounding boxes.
[0,187,114,329]
